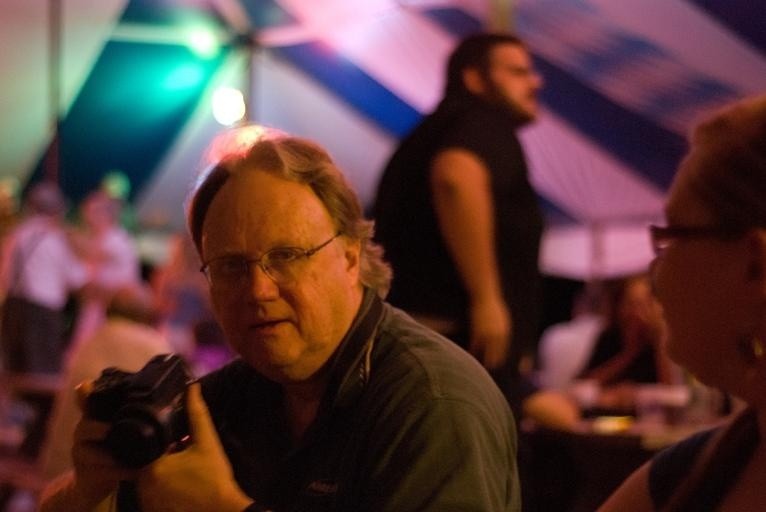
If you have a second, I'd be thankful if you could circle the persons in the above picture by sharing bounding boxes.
[33,137,524,511]
[594,91,764,512]
[2,173,735,512]
[363,30,545,387]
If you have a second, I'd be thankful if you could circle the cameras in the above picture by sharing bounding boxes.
[81,350,197,469]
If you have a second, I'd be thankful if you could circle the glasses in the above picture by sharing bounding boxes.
[643,222,747,260]
[197,225,344,293]
